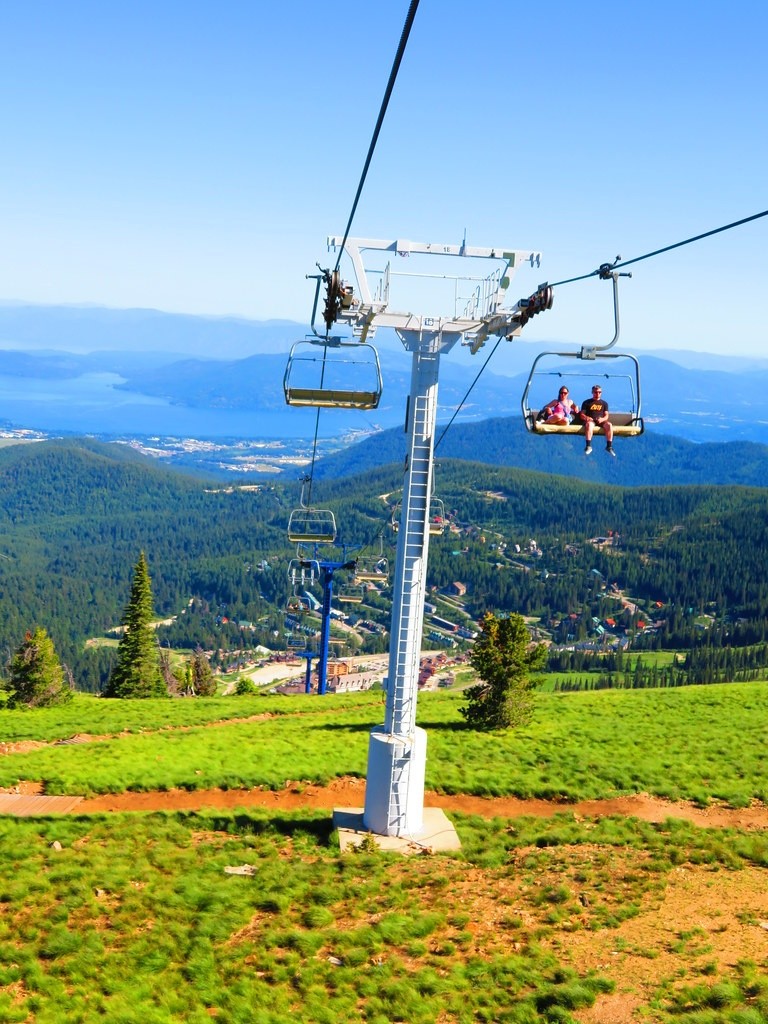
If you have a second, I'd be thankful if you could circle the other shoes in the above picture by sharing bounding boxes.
[584,445,593,454]
[605,446,616,456]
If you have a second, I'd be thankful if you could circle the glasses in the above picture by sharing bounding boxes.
[559,391,567,395]
[594,391,601,394]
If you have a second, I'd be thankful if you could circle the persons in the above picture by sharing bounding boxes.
[579,384,617,456]
[535,386,579,426]
